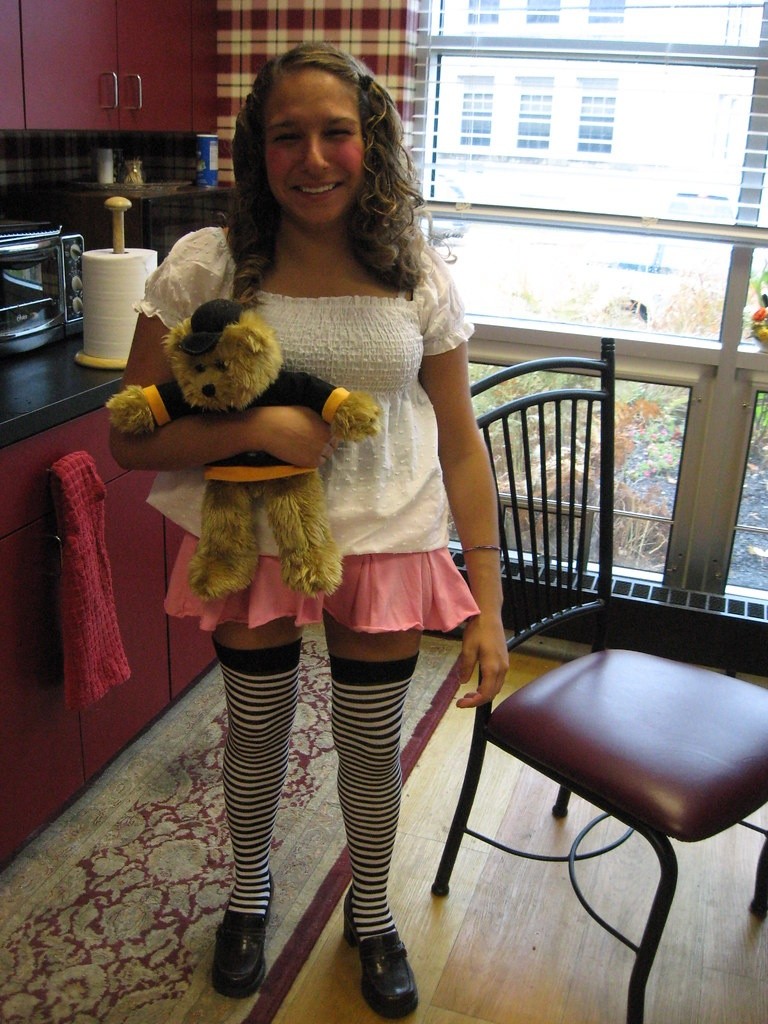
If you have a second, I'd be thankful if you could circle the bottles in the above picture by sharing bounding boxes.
[97,147,143,185]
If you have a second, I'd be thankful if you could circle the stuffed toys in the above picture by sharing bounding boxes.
[110,298,381,599]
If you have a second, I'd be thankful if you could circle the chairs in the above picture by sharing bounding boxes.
[431,338,768,1024]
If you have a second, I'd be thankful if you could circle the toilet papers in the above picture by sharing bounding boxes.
[81,244,157,357]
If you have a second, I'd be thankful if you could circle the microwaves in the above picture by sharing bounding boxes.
[1,232,85,354]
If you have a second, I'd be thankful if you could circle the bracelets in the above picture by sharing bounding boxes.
[463,545,501,558]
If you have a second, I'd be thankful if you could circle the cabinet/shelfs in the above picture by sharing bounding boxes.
[19,0,193,139]
[0,406,171,874]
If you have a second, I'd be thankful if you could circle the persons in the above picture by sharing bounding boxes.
[109,47,509,1018]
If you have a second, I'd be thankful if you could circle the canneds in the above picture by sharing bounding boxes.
[195,134,219,188]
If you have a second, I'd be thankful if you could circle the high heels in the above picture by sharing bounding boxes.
[211,873,274,999]
[343,883,418,1018]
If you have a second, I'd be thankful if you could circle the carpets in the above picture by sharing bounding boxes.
[0,623,465,1024]
